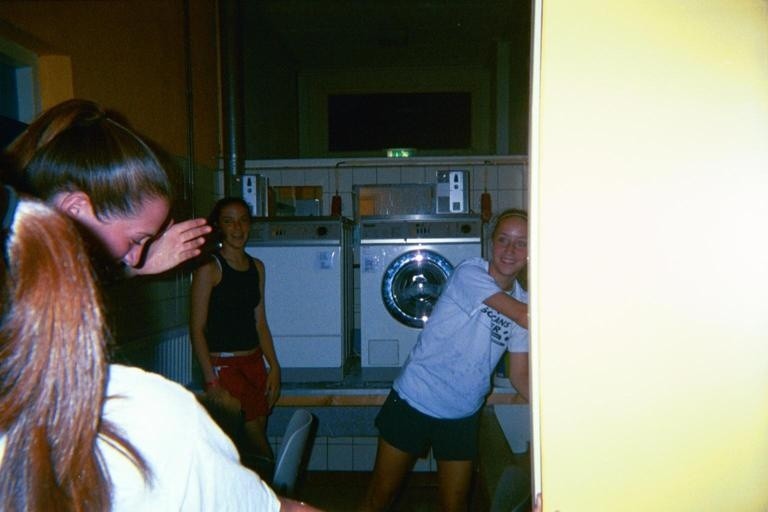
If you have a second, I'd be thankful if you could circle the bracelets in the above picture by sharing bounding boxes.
[202,379,220,389]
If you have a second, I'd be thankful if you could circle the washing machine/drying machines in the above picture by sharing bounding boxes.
[241,220,344,382]
[360,215,484,369]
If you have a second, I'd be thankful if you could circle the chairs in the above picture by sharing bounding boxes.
[234,406,313,501]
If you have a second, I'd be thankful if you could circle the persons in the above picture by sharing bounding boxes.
[358,209,530,512]
[0,185,324,512]
[1,100,213,279]
[189,197,282,469]
